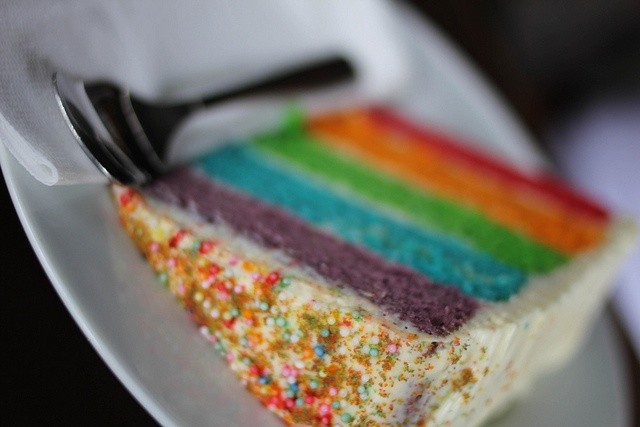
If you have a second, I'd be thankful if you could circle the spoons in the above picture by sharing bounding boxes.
[51,58,355,186]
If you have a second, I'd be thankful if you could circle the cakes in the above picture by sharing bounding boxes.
[111,105,637,427]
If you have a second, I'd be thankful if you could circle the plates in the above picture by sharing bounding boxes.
[0,1,633,427]
